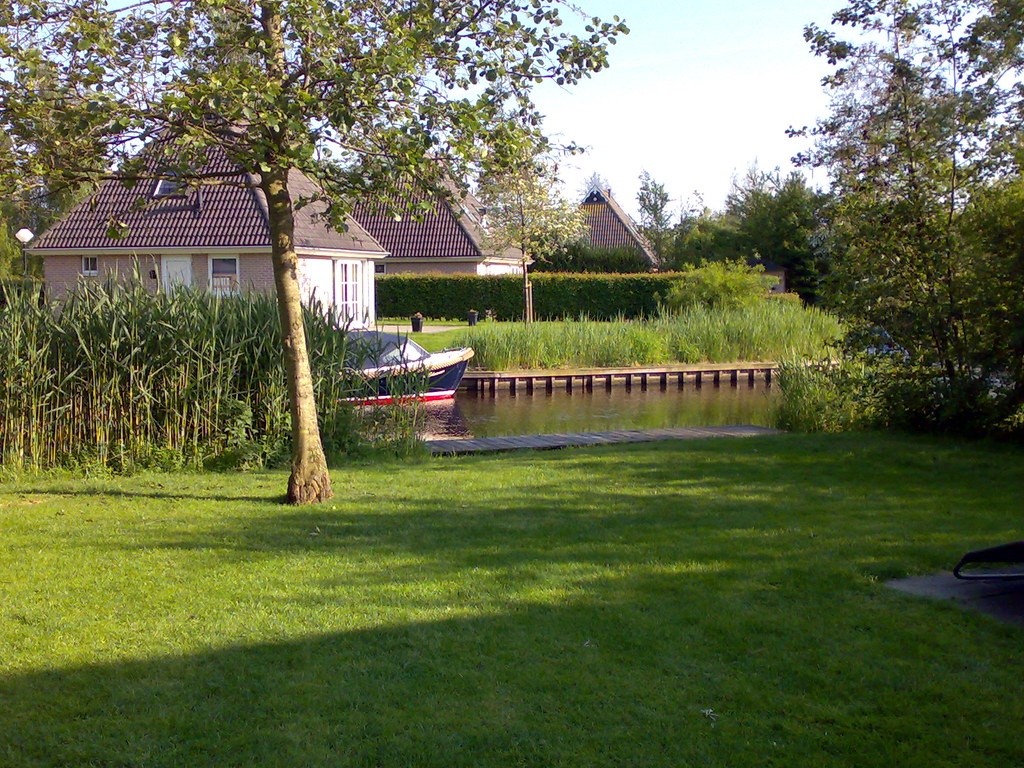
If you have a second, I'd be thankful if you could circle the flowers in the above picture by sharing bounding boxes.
[412,312,422,318]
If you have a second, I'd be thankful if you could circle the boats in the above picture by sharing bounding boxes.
[224,324,478,409]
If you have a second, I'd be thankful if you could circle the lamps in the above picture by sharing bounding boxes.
[149,270,157,280]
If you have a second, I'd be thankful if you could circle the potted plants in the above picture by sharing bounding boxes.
[468,309,478,326]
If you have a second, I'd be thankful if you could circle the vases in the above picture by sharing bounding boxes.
[411,318,423,332]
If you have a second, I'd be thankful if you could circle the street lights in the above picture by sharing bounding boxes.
[14,226,35,274]
